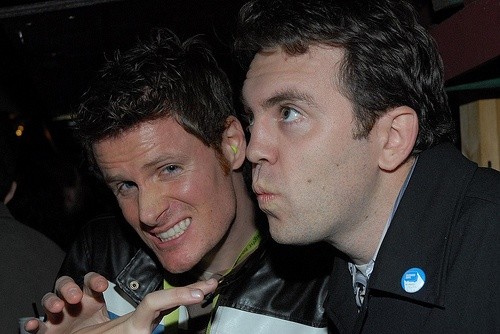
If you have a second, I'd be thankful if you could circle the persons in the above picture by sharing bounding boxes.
[23,33,332,334]
[241,0,500,333]
[1,152,67,333]
